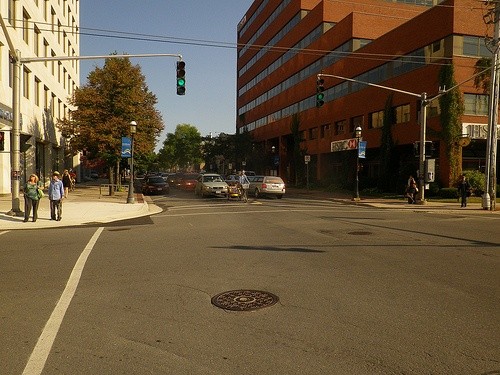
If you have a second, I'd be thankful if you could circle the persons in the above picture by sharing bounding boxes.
[238,169,250,200]
[405,175,418,204]
[62,168,76,198]
[48,171,64,221]
[458,176,472,207]
[23,174,44,223]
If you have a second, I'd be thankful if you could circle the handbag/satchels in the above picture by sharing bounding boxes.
[38,181,45,199]
[465,189,472,197]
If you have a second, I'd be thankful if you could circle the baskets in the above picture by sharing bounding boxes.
[242,184,249,189]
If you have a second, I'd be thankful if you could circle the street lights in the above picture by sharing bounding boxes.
[127,118,137,204]
[353,126,362,201]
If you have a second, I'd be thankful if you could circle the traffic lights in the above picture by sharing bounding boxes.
[0,132,4,151]
[316,78,325,108]
[176,61,186,95]
[425,141,440,157]
[19,134,33,153]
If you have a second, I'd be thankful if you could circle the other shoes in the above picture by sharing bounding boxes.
[33,220,36,222]
[24,219,28,222]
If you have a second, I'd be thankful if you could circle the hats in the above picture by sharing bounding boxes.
[53,171,60,174]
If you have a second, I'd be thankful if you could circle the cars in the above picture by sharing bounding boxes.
[136,170,168,195]
[167,172,230,200]
[225,170,286,199]
